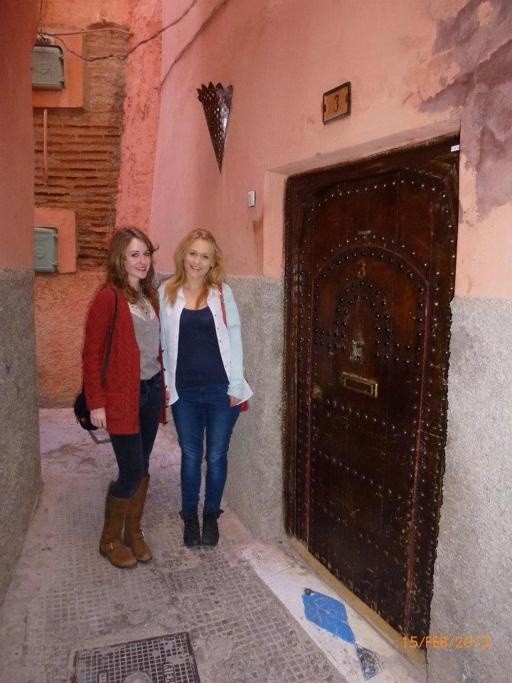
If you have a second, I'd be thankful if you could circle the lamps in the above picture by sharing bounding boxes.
[195,78,234,171]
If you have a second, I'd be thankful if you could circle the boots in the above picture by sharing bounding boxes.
[124,474,152,561]
[202,508,224,546]
[100,481,137,568]
[179,509,201,546]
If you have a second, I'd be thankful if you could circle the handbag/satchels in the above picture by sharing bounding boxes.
[73,393,102,431]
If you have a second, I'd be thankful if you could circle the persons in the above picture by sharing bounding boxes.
[82,226,169,569]
[157,225,253,548]
